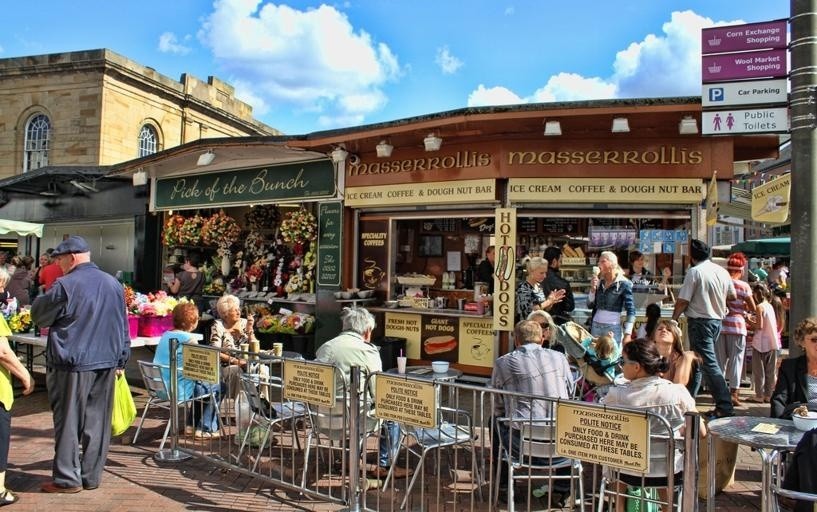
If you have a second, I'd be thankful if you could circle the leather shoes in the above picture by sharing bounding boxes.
[40,480,83,493]
[373,464,414,478]
[492,487,516,504]
[551,487,579,508]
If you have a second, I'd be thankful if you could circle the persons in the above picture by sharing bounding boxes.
[210,295,275,419]
[475,237,817,512]
[30,235,131,492]
[0,249,64,304]
[150,302,223,440]
[166,252,206,316]
[0,270,36,508]
[312,300,414,478]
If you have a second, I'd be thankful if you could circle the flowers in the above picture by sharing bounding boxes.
[5,307,33,332]
[155,205,316,335]
[116,286,196,316]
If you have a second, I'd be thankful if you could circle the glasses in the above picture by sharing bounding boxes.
[617,357,635,367]
[805,335,817,343]
[540,320,549,330]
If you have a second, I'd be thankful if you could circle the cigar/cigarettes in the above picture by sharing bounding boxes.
[250,314,256,316]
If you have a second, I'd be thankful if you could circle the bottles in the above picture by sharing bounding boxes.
[441,270,456,289]
[476,294,492,315]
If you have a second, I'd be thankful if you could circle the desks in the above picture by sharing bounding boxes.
[7,331,206,446]
[707,416,817,511]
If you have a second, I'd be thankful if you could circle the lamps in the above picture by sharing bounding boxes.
[609,113,632,135]
[542,116,562,135]
[130,166,148,186]
[196,148,216,165]
[422,133,443,152]
[677,112,699,135]
[373,135,394,158]
[331,147,348,161]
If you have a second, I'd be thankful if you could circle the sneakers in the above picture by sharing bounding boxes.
[703,408,733,418]
[193,429,223,440]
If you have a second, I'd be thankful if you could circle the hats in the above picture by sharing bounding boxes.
[691,236,710,259]
[51,235,89,256]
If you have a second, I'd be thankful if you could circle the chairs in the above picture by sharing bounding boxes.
[492,413,585,512]
[595,431,700,511]
[136,346,491,510]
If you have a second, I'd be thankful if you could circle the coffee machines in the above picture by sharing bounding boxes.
[460,269,473,290]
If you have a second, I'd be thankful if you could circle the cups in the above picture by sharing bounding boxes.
[240,343,248,359]
[250,340,260,360]
[396,356,407,374]
[272,343,282,357]
[456,298,464,310]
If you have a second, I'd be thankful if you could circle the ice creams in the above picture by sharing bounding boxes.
[754,194,784,217]
[662,267,671,282]
[593,266,600,278]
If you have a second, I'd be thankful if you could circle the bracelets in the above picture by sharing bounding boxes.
[245,329,253,336]
[229,356,236,363]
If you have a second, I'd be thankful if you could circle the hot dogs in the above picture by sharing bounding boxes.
[496,246,513,279]
[424,335,457,354]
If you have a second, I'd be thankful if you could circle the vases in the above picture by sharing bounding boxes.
[17,321,32,333]
[124,314,174,339]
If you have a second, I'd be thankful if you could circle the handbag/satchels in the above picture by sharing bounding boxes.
[625,485,658,512]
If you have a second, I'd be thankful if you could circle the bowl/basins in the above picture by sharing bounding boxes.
[431,361,449,374]
[790,411,816,432]
[385,301,398,309]
[334,290,374,299]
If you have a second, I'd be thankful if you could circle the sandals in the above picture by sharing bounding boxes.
[185,427,194,438]
[0,488,18,506]
[730,391,770,406]
